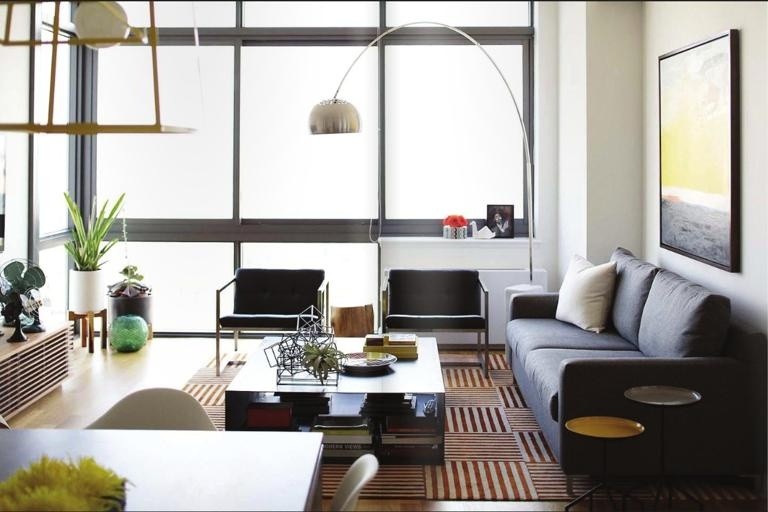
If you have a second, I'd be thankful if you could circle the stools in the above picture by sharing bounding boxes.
[65,309,107,355]
[622,383,701,510]
[562,414,647,510]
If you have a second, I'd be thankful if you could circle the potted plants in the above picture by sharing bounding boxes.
[60,193,128,314]
[104,265,151,347]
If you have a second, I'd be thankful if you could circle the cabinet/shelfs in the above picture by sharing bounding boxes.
[1,322,77,424]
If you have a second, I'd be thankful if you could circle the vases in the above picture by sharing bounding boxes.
[441,223,467,238]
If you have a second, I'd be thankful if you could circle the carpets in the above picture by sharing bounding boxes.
[181,351,759,502]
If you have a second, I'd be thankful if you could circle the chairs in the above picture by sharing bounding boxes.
[83,388,219,431]
[381,268,489,381]
[317,452,383,511]
[214,268,328,376]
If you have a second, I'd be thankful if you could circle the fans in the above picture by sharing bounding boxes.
[1,258,54,330]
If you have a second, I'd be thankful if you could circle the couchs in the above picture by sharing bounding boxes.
[505,244,765,492]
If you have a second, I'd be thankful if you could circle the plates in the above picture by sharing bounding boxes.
[333,352,398,371]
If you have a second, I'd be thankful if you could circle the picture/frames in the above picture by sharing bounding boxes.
[656,27,742,275]
[485,204,513,239]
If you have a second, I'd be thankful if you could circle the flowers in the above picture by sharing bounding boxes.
[1,455,133,511]
[443,213,467,226]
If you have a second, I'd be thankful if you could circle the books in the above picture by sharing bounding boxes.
[363,332,419,358]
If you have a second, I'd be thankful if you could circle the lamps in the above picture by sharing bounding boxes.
[308,19,536,286]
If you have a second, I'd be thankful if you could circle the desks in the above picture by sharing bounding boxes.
[0,430,327,511]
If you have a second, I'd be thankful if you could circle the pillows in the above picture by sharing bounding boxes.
[553,254,616,336]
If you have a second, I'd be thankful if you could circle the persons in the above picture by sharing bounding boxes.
[246,391,440,457]
[491,213,511,235]
